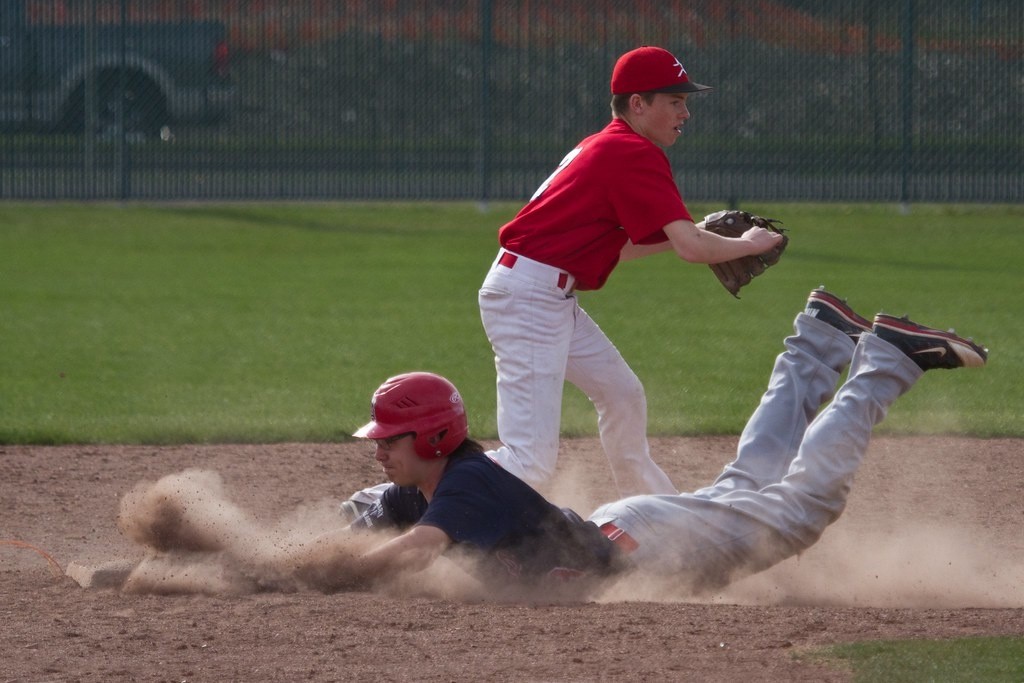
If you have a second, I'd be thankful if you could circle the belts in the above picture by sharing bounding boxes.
[600,523,638,552]
[500,253,568,289]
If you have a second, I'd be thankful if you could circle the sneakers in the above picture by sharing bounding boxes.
[872,312,989,373]
[804,285,874,342]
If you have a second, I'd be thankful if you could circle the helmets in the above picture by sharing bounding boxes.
[351,369,468,459]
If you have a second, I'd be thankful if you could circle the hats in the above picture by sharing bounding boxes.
[610,45,713,94]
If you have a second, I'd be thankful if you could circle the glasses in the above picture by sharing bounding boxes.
[368,431,413,451]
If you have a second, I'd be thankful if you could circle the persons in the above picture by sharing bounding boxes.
[294,288,989,594]
[336,44,791,526]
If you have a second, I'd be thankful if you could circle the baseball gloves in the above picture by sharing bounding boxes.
[700,208,794,301]
